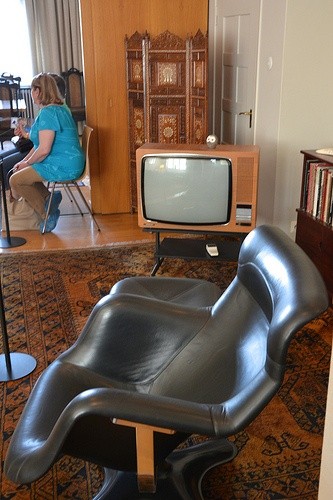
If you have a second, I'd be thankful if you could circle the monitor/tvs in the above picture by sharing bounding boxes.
[135,142,260,233]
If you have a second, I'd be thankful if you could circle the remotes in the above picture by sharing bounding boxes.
[205,243,219,257]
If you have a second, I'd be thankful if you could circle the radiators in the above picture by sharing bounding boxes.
[19,87,34,119]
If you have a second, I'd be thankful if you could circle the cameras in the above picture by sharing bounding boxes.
[10,117,18,129]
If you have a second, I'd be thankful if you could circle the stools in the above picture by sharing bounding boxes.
[110,276,222,307]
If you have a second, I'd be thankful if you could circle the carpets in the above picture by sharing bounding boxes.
[0,235,333,500]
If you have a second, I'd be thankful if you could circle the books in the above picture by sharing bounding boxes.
[301,160,333,227]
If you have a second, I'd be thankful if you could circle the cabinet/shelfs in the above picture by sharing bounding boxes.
[296,148,333,307]
[142,228,249,276]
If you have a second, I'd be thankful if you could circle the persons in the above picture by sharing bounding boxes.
[9,73,85,232]
[0,74,66,190]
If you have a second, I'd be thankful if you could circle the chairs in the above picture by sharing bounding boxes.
[61,67,86,136]
[41,124,101,234]
[4,225,330,500]
[0,72,22,151]
